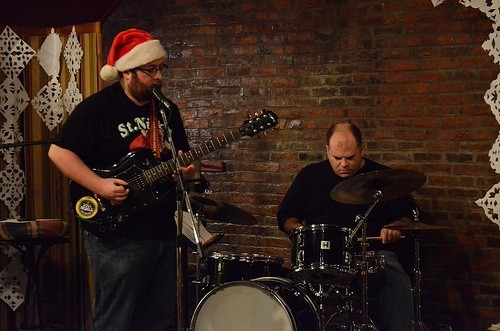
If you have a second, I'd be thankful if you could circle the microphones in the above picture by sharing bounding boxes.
[149,84,172,110]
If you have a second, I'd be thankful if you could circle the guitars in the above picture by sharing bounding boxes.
[66,108,280,227]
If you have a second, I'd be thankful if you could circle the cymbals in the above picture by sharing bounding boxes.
[182,195,258,226]
[330,168,427,205]
[384,222,452,232]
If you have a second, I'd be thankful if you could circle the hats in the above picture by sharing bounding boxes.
[100,28,167,81]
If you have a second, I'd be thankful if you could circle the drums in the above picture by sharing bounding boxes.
[205,251,283,289]
[187,276,321,331]
[289,224,359,285]
[354,253,386,275]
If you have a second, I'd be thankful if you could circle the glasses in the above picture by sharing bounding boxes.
[138,65,168,76]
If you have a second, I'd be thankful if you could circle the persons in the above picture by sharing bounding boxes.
[277,121,424,331]
[48,28,196,331]
